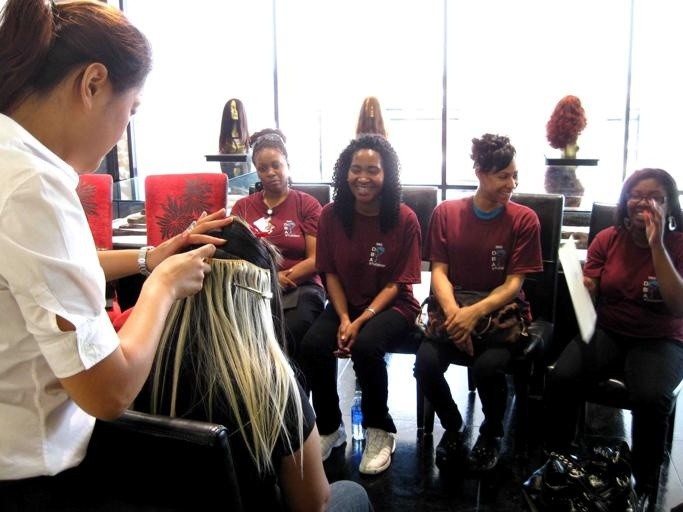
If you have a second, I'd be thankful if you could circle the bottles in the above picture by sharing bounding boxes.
[352,390,366,442]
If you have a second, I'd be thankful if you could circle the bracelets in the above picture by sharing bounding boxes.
[137,246,154,277]
[365,308,376,315]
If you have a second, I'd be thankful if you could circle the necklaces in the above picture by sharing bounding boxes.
[267,209,273,214]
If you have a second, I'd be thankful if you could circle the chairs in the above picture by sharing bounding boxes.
[94,410,239,512]
[77,174,122,322]
[426,194,564,433]
[249,185,329,209]
[385,188,436,439]
[110,172,228,333]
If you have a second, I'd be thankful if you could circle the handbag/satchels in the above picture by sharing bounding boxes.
[413,290,534,349]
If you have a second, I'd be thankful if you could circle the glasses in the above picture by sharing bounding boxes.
[623,191,672,206]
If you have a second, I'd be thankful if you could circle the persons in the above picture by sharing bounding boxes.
[302,134,423,475]
[413,134,544,472]
[226,129,324,411]
[125,215,372,512]
[0,0,234,512]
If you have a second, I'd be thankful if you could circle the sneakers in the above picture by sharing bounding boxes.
[313,416,348,463]
[435,422,471,464]
[357,423,397,476]
[465,428,506,474]
